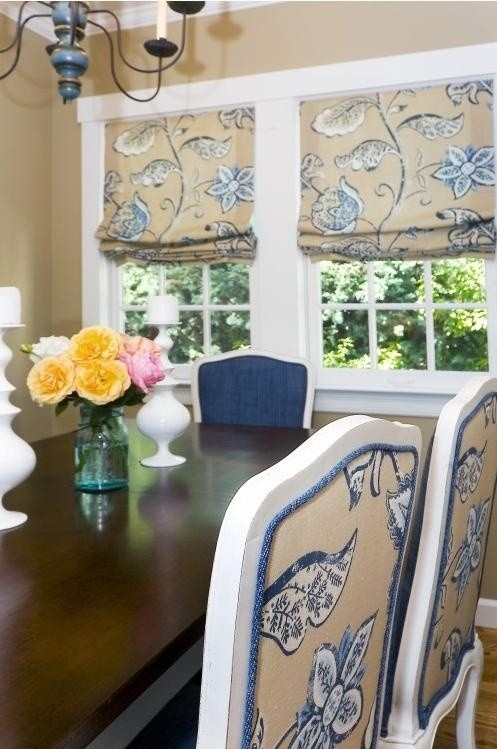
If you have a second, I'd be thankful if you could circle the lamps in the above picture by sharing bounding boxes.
[0,1,207,103]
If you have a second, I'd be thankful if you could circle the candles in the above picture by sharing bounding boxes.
[0,286,23,324]
[146,294,180,322]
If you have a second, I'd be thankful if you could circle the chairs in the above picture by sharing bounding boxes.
[374,370,496,748]
[188,411,425,746]
[188,348,318,431]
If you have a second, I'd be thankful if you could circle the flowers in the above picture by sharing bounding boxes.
[18,324,165,472]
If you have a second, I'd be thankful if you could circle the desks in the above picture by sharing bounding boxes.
[0,403,439,747]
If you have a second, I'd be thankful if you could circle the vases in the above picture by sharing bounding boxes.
[73,400,132,490]
